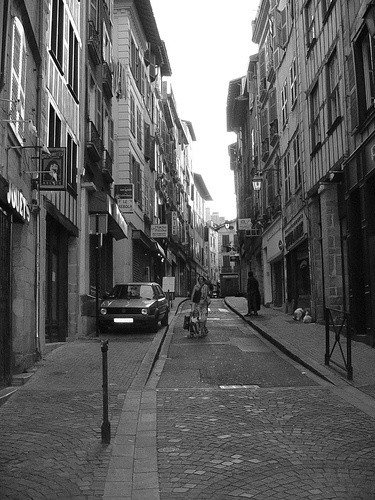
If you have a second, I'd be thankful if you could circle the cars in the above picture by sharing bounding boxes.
[99,282,169,333]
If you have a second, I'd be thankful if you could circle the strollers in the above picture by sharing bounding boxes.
[185,304,206,337]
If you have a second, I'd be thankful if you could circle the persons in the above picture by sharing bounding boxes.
[187,276,211,338]
[244,271,261,317]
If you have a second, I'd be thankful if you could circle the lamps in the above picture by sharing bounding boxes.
[251,172,263,192]
[224,219,231,230]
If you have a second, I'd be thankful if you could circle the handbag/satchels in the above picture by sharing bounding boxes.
[192,284,205,303]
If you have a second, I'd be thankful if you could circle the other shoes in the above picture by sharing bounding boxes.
[244,312,258,317]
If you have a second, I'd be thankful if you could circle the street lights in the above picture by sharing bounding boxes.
[252,174,262,191]
